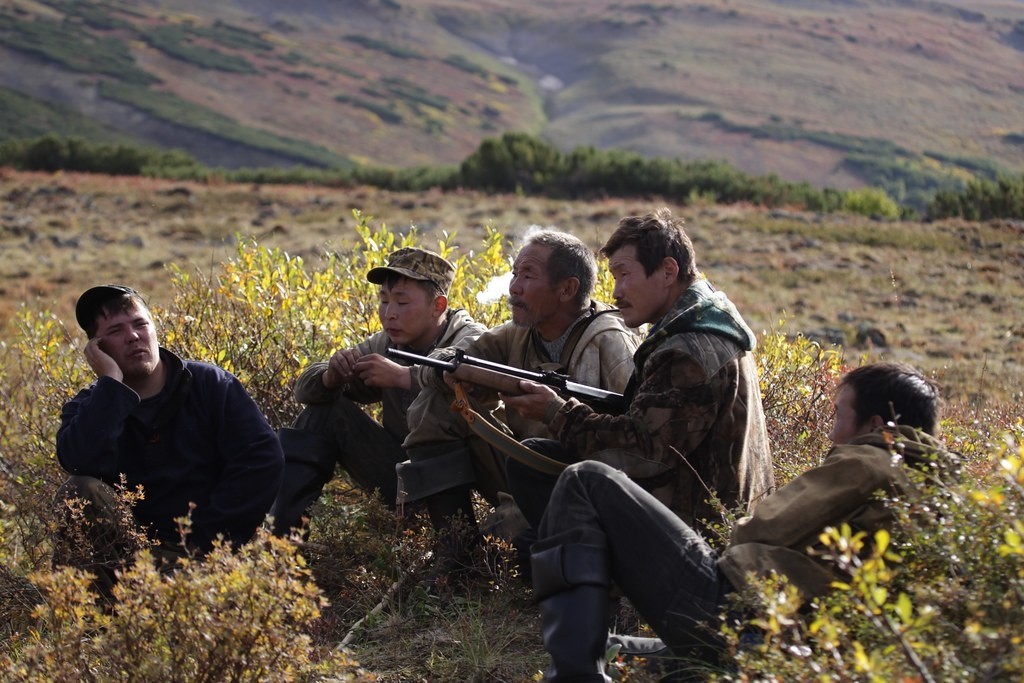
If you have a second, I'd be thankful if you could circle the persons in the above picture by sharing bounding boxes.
[497,206,775,583]
[53,284,286,634]
[267,248,490,538]
[528,358,966,683]
[397,228,641,598]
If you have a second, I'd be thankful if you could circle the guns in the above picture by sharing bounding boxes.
[385,346,627,413]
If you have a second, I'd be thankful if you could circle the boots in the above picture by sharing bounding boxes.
[395,439,481,579]
[606,634,674,671]
[269,427,337,564]
[529,527,612,683]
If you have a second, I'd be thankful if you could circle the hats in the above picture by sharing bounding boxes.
[76,284,152,331]
[367,246,457,298]
